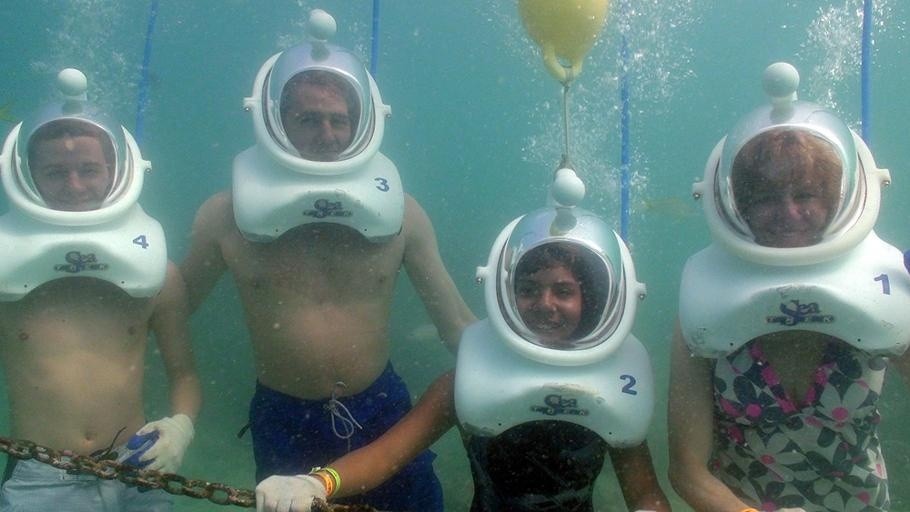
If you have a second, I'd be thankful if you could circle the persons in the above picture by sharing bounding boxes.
[0,117,209,512]
[255,240,672,512]
[664,125,908,512]
[168,69,487,512]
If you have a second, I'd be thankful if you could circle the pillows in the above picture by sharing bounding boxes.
[693,63,892,269]
[242,11,394,176]
[0,67,153,229]
[476,168,646,368]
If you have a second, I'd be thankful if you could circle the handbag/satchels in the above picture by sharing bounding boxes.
[323,463,341,500]
[309,469,332,497]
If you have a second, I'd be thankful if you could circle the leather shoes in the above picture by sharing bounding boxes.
[114,414,196,493]
[254,472,330,511]
